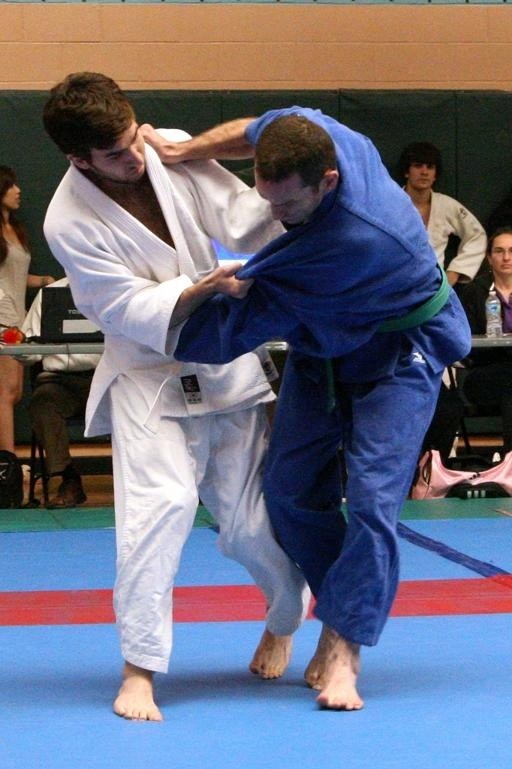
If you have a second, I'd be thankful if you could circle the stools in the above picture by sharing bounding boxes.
[28,384,113,504]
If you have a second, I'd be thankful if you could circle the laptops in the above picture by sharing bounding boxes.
[31,287,104,343]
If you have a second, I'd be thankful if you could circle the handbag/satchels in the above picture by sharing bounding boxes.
[0,449,23,508]
[410,449,512,500]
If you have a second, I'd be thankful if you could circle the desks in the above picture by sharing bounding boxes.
[0,337,512,355]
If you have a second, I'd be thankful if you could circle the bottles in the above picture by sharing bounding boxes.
[484,290,503,339]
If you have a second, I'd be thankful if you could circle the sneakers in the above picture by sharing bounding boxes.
[50,478,87,508]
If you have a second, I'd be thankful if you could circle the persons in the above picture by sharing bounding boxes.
[418,226,512,461]
[0,275,105,510]
[39,71,313,723]
[0,161,58,455]
[137,104,473,712]
[396,140,488,291]
